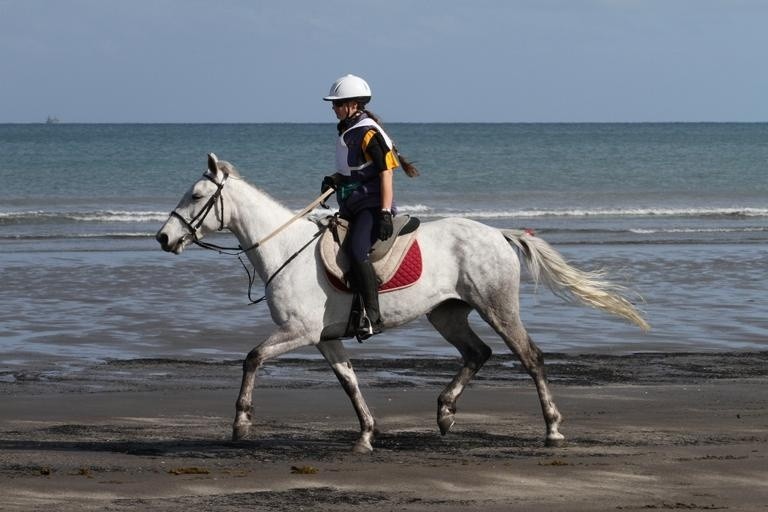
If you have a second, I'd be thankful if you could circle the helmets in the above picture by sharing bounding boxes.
[323,74,371,102]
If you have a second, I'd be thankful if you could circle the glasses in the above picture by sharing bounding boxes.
[331,100,348,106]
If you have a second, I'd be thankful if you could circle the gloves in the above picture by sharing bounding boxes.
[321,176,338,194]
[376,211,393,241]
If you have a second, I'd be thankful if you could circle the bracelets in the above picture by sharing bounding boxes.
[381,208,391,214]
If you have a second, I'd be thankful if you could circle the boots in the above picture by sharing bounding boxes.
[357,271,384,340]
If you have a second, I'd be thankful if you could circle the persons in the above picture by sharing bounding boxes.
[322,73,420,341]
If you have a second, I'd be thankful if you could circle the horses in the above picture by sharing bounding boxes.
[155,152,651,455]
